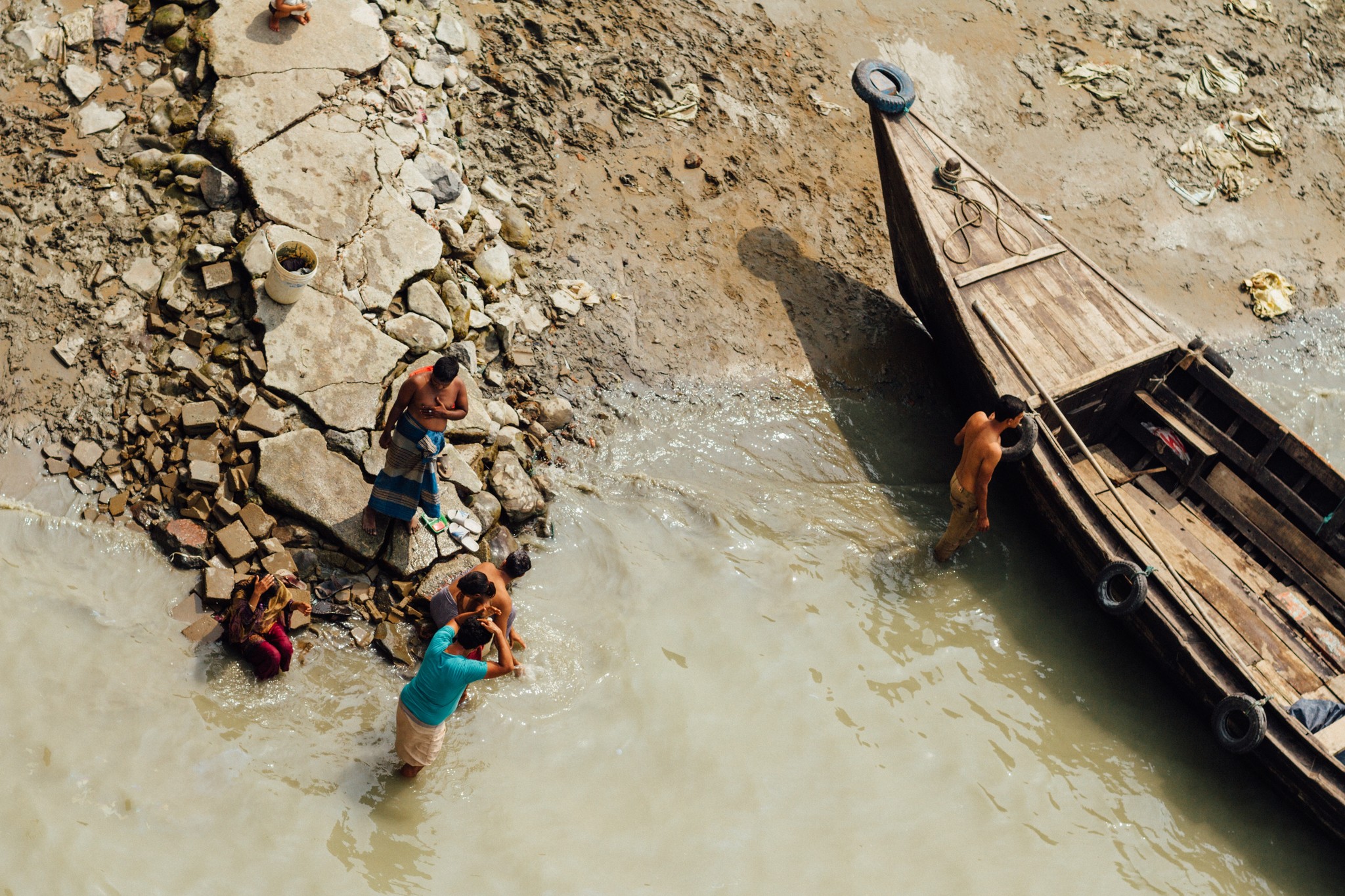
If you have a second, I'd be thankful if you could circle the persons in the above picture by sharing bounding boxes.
[395,606,514,780]
[455,571,496,706]
[430,548,532,679]
[227,572,313,680]
[361,354,470,538]
[933,395,1025,564]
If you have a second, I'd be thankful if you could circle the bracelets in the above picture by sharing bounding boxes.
[248,601,257,609]
[289,601,295,611]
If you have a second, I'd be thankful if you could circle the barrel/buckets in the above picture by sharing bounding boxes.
[265,240,319,304]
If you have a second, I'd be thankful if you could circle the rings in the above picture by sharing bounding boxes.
[429,410,432,413]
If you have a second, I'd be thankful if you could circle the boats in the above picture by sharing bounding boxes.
[851,59,1345,844]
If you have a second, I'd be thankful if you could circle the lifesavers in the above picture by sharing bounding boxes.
[1187,337,1234,379]
[1210,693,1267,755]
[1000,412,1038,464]
[1094,561,1148,619]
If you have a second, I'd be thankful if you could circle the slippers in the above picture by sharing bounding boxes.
[313,576,352,599]
[276,569,308,590]
[447,509,482,534]
[449,522,480,553]
[311,602,353,620]
